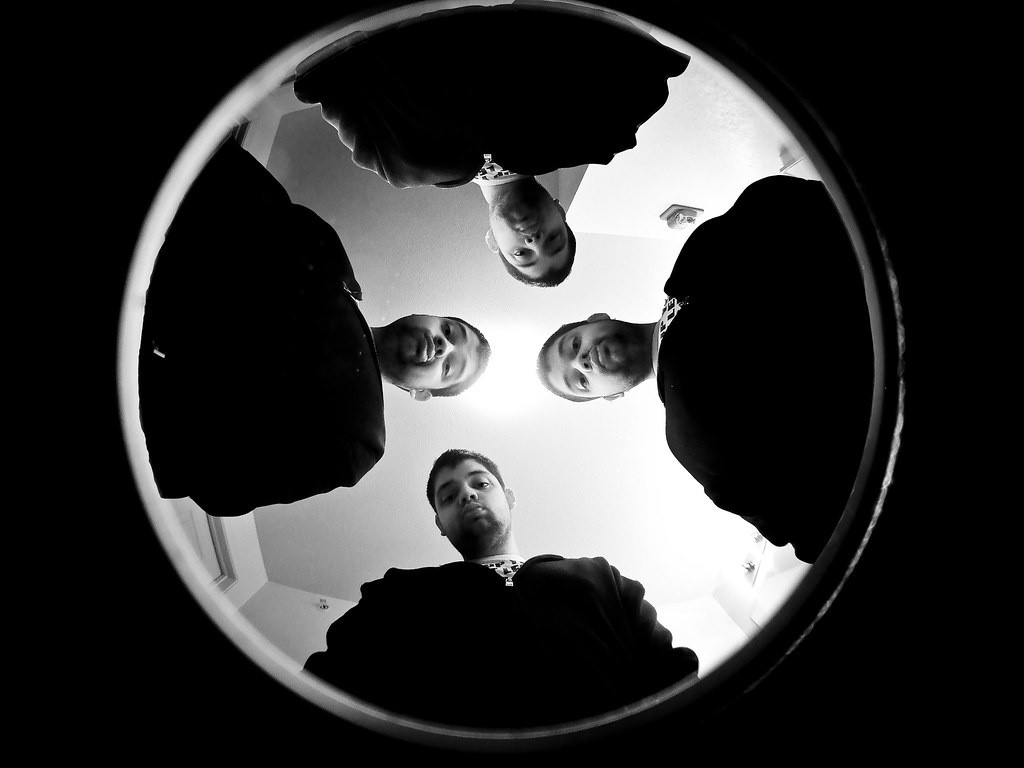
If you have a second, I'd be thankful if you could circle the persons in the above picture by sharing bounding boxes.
[135,136,491,519]
[301,448,700,734]
[292,5,692,288]
[537,173,868,570]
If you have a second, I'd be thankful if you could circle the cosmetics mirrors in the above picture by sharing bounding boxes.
[116,0,905,752]
